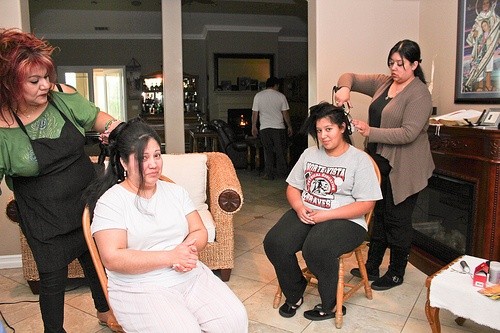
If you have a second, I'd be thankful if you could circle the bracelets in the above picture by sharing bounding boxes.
[104,119,117,131]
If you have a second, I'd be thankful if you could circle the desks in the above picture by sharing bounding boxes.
[188,129,219,153]
[425,255,500,333]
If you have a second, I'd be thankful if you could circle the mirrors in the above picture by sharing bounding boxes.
[214,52,275,90]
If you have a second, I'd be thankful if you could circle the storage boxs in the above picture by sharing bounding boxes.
[474,263,489,287]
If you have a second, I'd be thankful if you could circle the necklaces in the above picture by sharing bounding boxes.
[386,83,405,100]
[20,105,38,118]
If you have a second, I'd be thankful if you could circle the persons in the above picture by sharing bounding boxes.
[252,77,293,179]
[83,117,248,333]
[0,27,121,333]
[263,101,382,320]
[335,40,435,290]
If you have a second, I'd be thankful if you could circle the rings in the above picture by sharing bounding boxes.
[336,101,339,104]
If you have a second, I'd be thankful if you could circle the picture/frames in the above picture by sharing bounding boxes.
[454,0,500,104]
[479,108,500,126]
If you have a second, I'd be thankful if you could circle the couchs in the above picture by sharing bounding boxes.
[5,152,243,295]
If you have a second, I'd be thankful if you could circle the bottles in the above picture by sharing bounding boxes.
[460,261,470,273]
[489,261,500,284]
[143,81,196,114]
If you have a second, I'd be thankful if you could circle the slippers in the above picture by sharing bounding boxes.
[99,314,125,333]
[279,296,303,317]
[303,304,346,321]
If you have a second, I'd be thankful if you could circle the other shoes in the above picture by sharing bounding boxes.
[371,265,403,290]
[350,267,380,281]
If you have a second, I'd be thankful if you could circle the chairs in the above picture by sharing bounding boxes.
[82,176,176,333]
[209,118,248,169]
[273,154,382,329]
